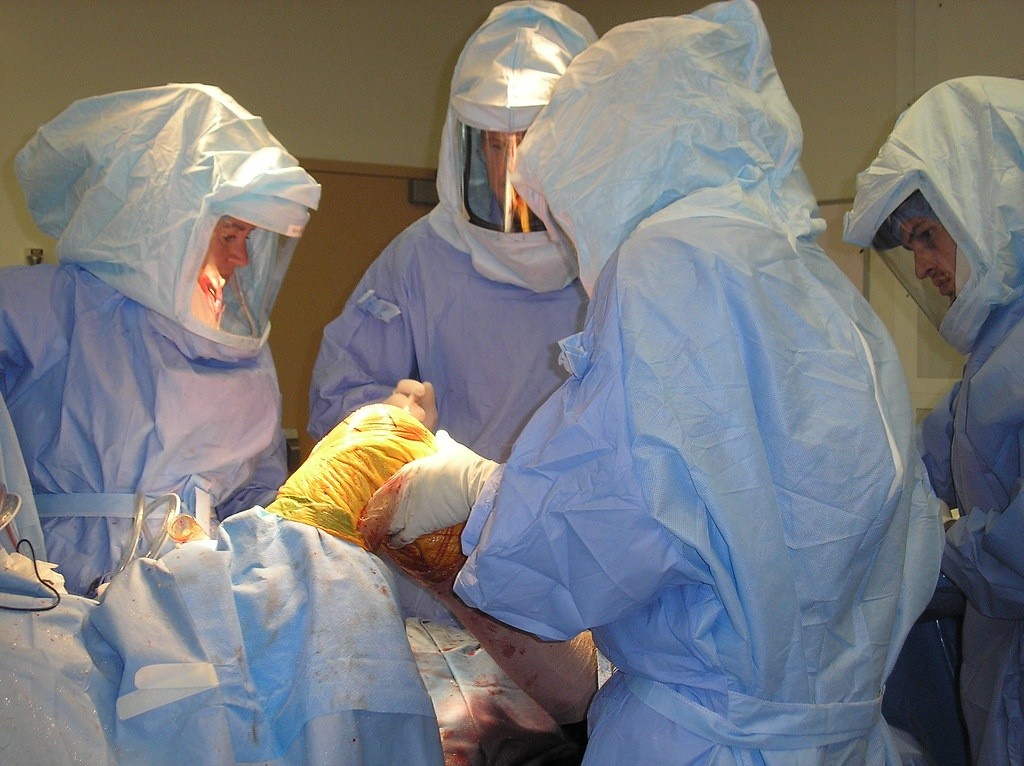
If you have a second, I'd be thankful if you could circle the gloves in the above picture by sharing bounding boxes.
[382,379,438,433]
[355,429,501,554]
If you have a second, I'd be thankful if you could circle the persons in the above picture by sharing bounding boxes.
[304,1,600,462]
[270,404,615,766]
[1,81,322,599]
[364,1,946,765]
[840,75,1024,766]
